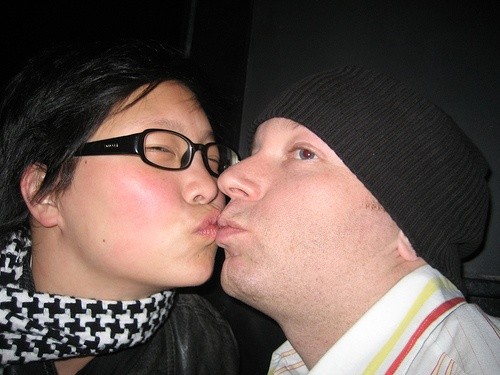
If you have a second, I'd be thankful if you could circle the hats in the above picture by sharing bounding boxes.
[246,63,492,302]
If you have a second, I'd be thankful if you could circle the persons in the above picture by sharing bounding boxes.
[216,68,500,375]
[0,36,239,375]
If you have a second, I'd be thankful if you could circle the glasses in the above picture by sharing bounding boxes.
[74,127,241,177]
